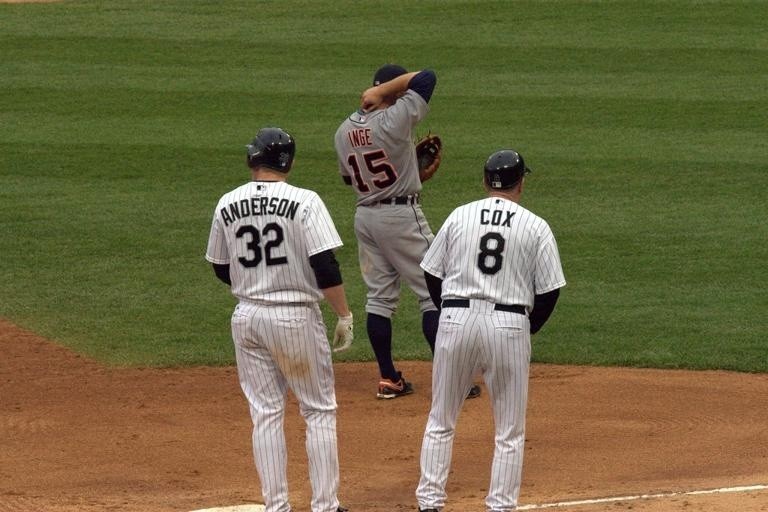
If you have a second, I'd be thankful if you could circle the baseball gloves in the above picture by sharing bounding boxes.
[415,135,442,182]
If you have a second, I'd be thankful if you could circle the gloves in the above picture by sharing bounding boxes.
[332,311,354,354]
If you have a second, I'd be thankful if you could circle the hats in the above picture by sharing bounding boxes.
[372,64,409,87]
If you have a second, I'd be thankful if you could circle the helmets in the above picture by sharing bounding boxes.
[482,149,533,190]
[247,127,295,173]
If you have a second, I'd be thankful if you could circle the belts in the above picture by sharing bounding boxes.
[361,196,420,205]
[442,298,526,313]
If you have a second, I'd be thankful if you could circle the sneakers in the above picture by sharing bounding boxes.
[466,385,481,399]
[376,378,415,398]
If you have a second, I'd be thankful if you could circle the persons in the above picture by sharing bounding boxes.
[203,124,354,512]
[333,66,481,398]
[414,150,566,512]
